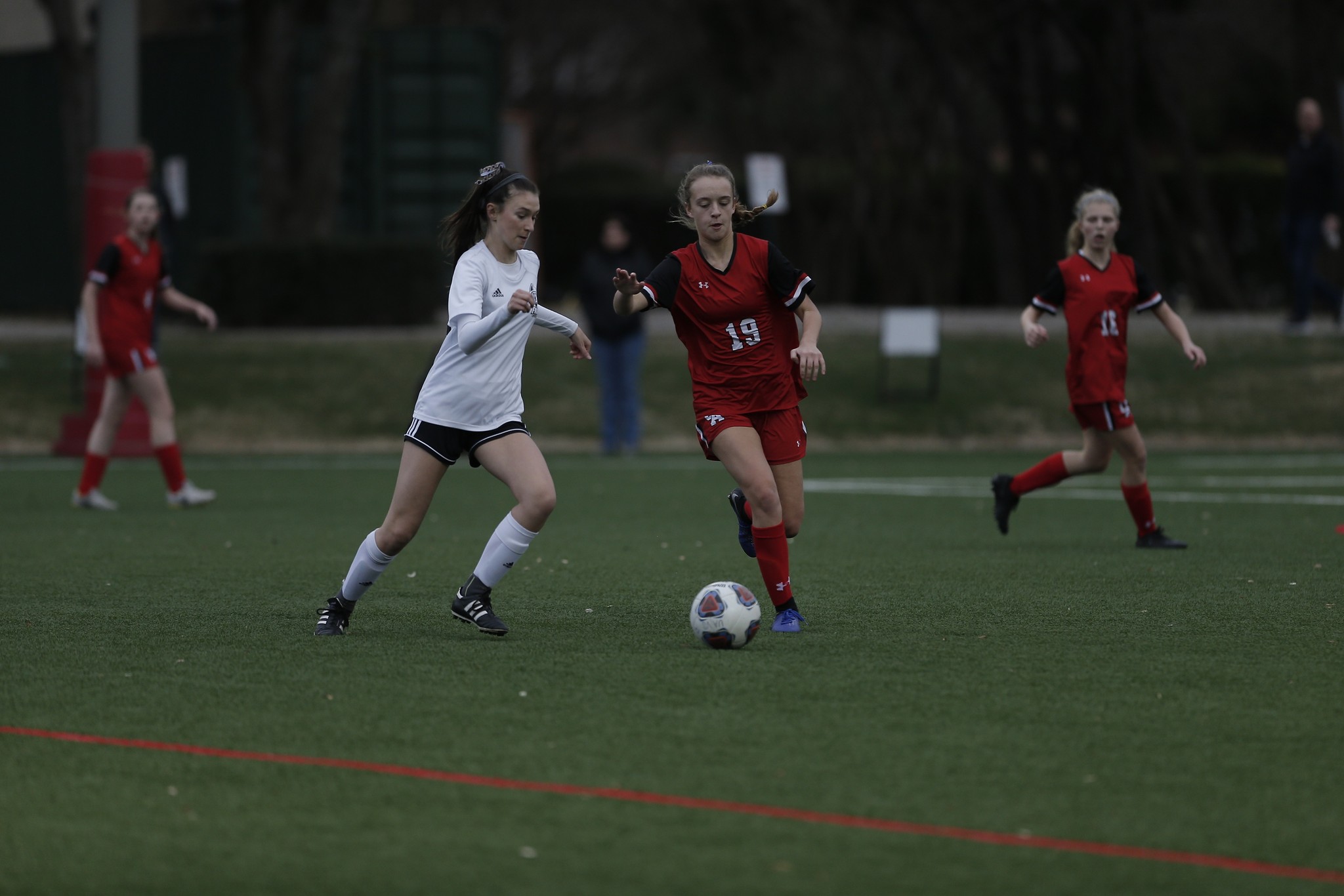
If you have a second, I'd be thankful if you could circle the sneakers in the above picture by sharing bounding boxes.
[771,610,802,633]
[167,481,216,508]
[728,490,757,557]
[1137,527,1185,549]
[451,586,509,634]
[992,473,1020,534]
[69,489,120,511]
[314,597,351,635]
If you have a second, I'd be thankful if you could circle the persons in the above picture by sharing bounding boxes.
[989,187,1208,550]
[1275,98,1344,336]
[75,189,227,516]
[314,160,594,638]
[576,210,652,458]
[611,159,828,635]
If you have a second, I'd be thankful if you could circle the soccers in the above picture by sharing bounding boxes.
[690,581,762,651]
